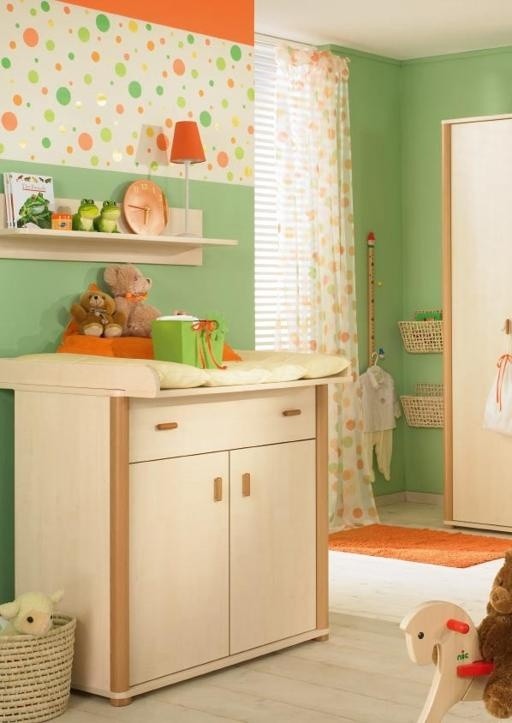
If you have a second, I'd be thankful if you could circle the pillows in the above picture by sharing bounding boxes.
[57,284,242,361]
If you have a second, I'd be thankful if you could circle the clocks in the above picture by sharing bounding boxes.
[123,180,170,235]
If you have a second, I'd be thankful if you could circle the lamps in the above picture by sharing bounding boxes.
[170,122,205,238]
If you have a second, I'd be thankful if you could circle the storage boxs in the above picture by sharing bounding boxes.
[151,315,224,369]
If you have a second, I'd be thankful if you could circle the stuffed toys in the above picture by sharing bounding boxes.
[73,197,100,230]
[101,263,187,338]
[93,198,121,232]
[431,549,512,718]
[69,280,127,337]
[1,588,69,637]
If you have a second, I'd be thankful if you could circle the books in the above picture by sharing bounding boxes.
[2,170,57,230]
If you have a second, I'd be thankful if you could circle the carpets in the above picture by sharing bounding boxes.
[328,525,512,568]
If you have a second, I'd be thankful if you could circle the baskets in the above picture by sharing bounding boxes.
[0,611,78,722]
[399,383,444,429]
[397,310,443,354]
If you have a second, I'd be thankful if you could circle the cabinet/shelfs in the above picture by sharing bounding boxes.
[0,376,353,708]
[441,113,511,533]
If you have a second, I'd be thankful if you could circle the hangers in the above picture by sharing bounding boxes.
[371,352,380,371]
